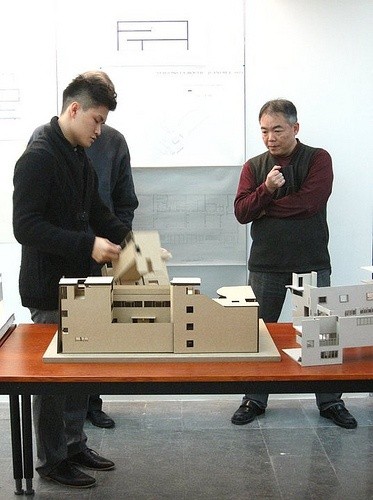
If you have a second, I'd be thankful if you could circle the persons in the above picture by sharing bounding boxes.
[12,72,139,488]
[230,98,359,430]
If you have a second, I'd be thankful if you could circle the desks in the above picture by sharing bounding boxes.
[0,323,373,495]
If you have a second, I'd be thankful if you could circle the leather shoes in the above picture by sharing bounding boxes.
[89,410,115,427]
[42,466,97,488]
[231,400,265,425]
[66,447,116,470]
[320,404,357,429]
